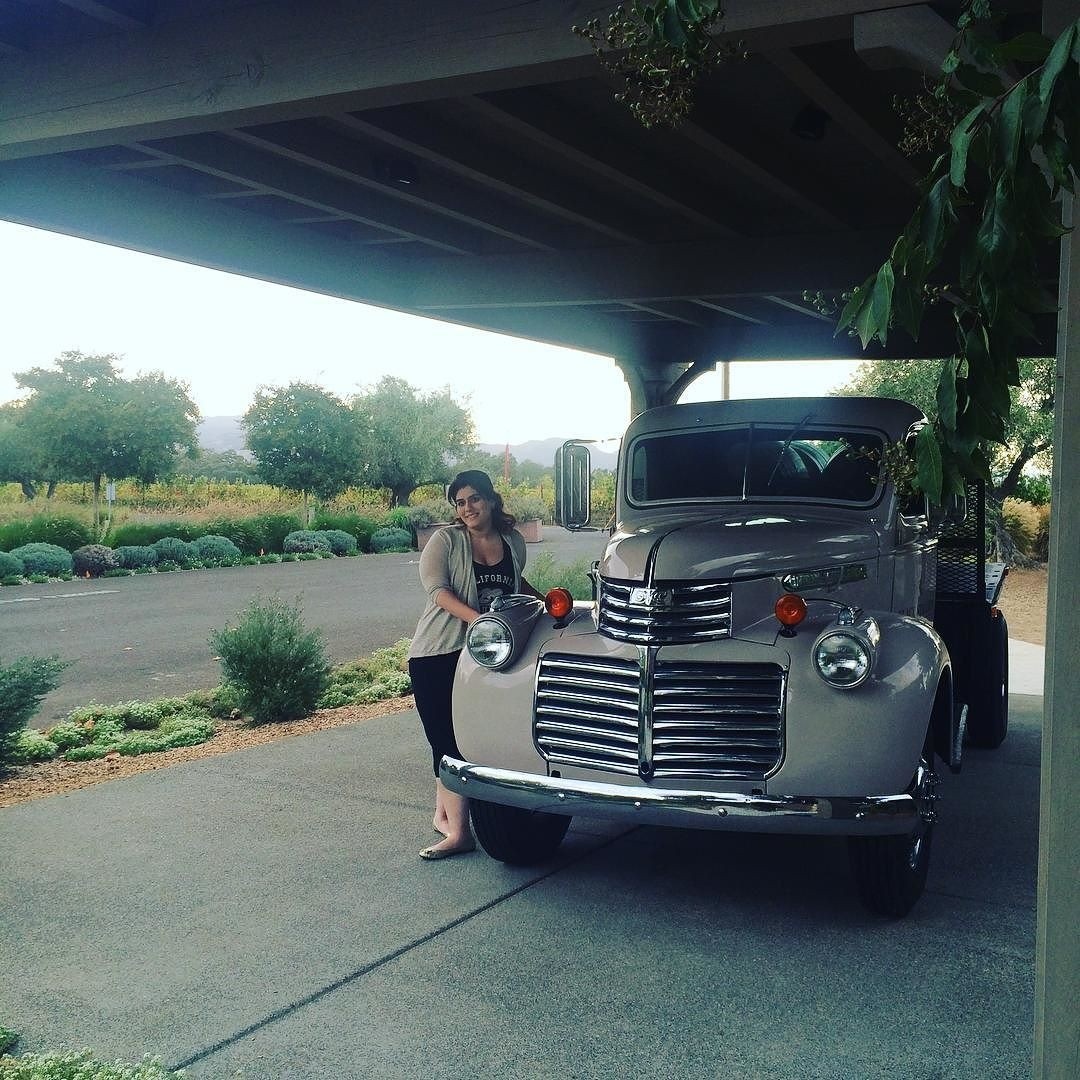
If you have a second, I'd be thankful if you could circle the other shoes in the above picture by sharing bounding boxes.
[419,837,476,860]
[432,816,451,840]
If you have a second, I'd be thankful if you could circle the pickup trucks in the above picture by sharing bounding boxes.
[440,397,1012,922]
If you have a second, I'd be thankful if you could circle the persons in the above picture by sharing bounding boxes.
[407,470,547,859]
